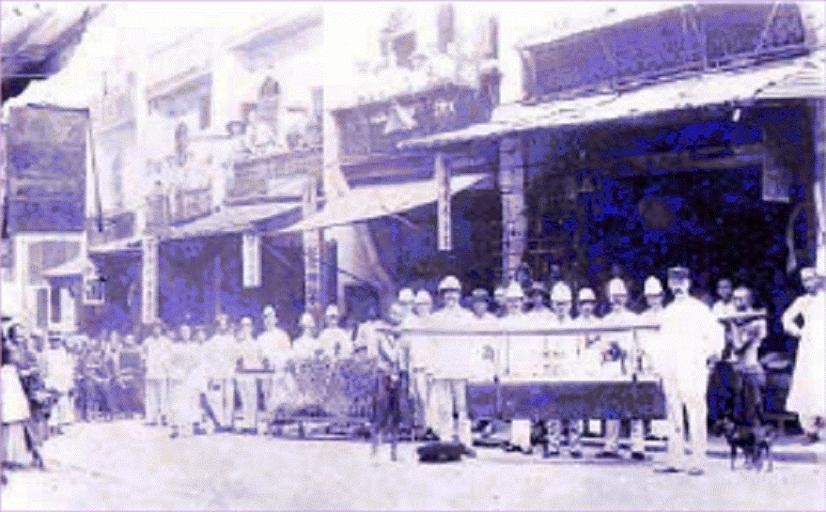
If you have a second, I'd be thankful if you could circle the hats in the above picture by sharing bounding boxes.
[48,330,62,340]
[668,267,690,278]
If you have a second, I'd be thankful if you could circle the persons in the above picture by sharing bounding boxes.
[2,264,773,487]
[781,266,826,444]
[146,38,506,199]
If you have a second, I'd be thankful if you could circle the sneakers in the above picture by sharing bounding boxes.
[504,441,709,476]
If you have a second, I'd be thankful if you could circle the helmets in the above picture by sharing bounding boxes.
[396,274,666,304]
[299,305,340,327]
